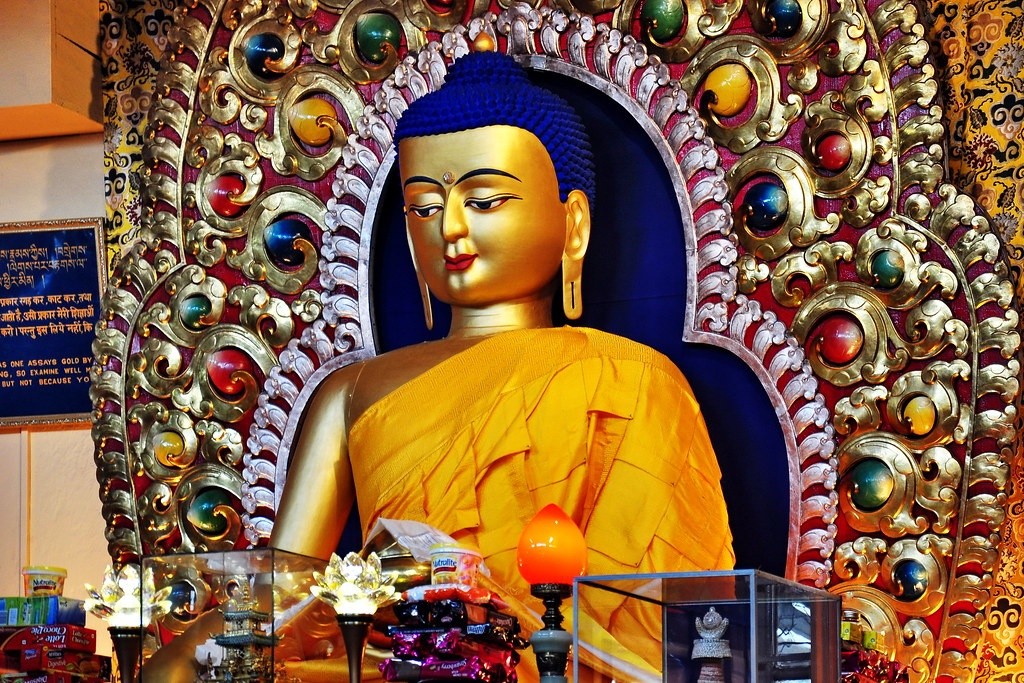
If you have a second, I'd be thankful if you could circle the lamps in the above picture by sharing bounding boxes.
[309,550,401,681]
[518,503,587,682]
[84,565,173,683]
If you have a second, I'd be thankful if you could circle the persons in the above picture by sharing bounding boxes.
[137,31,737,683]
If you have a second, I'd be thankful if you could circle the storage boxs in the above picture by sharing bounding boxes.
[0,594,112,683]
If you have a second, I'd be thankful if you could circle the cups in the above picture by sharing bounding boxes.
[21,565,67,598]
